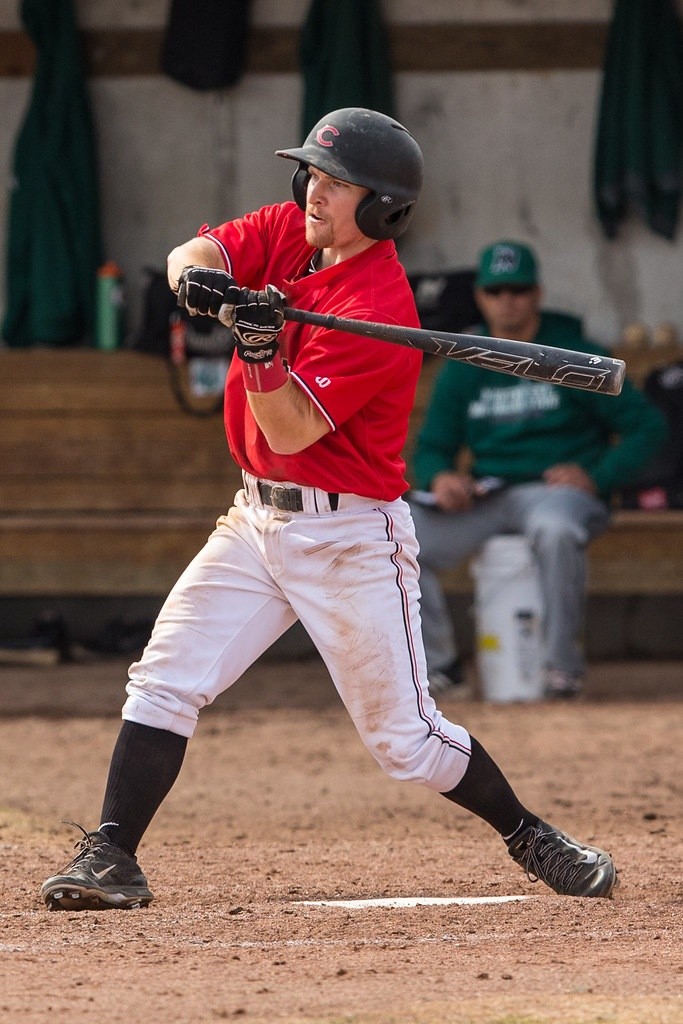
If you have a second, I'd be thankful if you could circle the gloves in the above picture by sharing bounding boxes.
[232,284,286,362]
[176,266,233,328]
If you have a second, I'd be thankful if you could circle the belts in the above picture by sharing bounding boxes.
[245,480,339,513]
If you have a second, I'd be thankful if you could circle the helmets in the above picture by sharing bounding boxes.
[274,106,425,239]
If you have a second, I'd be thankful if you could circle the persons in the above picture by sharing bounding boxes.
[402,237,673,699]
[40,108,615,913]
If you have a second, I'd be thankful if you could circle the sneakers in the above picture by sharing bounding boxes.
[38,820,154,911]
[508,817,616,899]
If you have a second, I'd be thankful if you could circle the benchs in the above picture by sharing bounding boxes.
[0,344,683,602]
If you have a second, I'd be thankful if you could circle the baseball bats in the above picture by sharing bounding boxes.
[174,278,628,396]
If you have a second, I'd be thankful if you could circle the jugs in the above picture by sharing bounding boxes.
[470,526,545,702]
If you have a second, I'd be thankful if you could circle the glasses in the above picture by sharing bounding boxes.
[485,284,531,297]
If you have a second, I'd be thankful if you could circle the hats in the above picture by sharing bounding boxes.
[474,239,539,290]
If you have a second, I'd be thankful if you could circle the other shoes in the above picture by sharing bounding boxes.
[424,661,466,696]
[544,673,586,700]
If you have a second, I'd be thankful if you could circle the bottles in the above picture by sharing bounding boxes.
[96,261,124,351]
